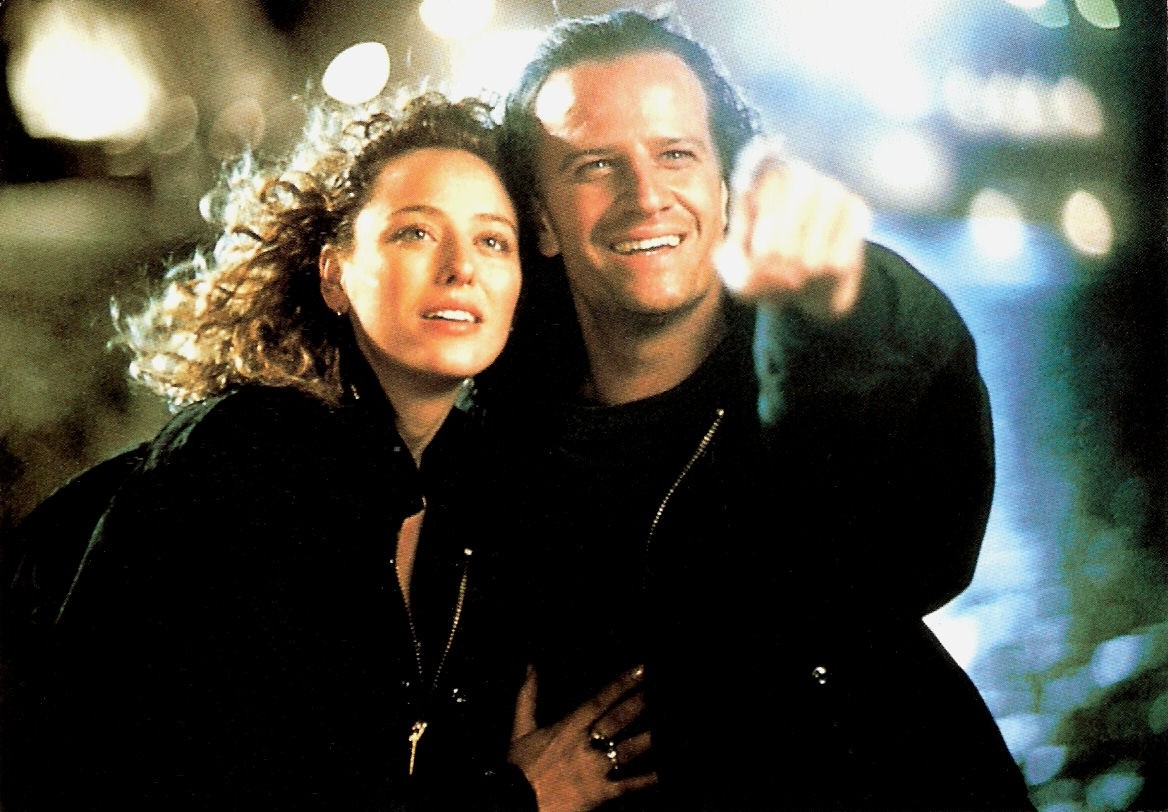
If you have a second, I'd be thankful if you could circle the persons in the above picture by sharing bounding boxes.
[0,6,1024,812]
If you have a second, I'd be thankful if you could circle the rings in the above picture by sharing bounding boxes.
[591,727,608,744]
[605,741,621,772]
